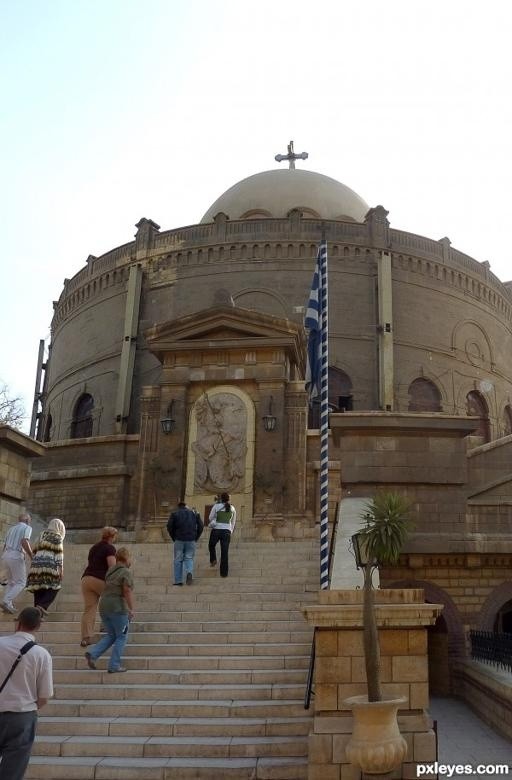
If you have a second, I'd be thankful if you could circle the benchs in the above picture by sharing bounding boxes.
[303,247,322,403]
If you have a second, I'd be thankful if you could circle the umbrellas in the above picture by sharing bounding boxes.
[109,666,128,673]
[186,573,192,585]
[174,583,183,585]
[80,639,92,647]
[85,651,97,670]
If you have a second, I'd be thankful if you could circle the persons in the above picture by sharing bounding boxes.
[24,518,66,618]
[85,547,136,673]
[0,513,33,614]
[167,502,204,586]
[80,526,118,646]
[0,608,54,780]
[208,493,236,578]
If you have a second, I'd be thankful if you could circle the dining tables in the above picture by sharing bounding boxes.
[341,490,415,774]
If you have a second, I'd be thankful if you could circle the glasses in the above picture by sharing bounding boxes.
[262,415,276,432]
[161,417,176,434]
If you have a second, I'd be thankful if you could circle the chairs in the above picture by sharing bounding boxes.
[1,598,17,615]
